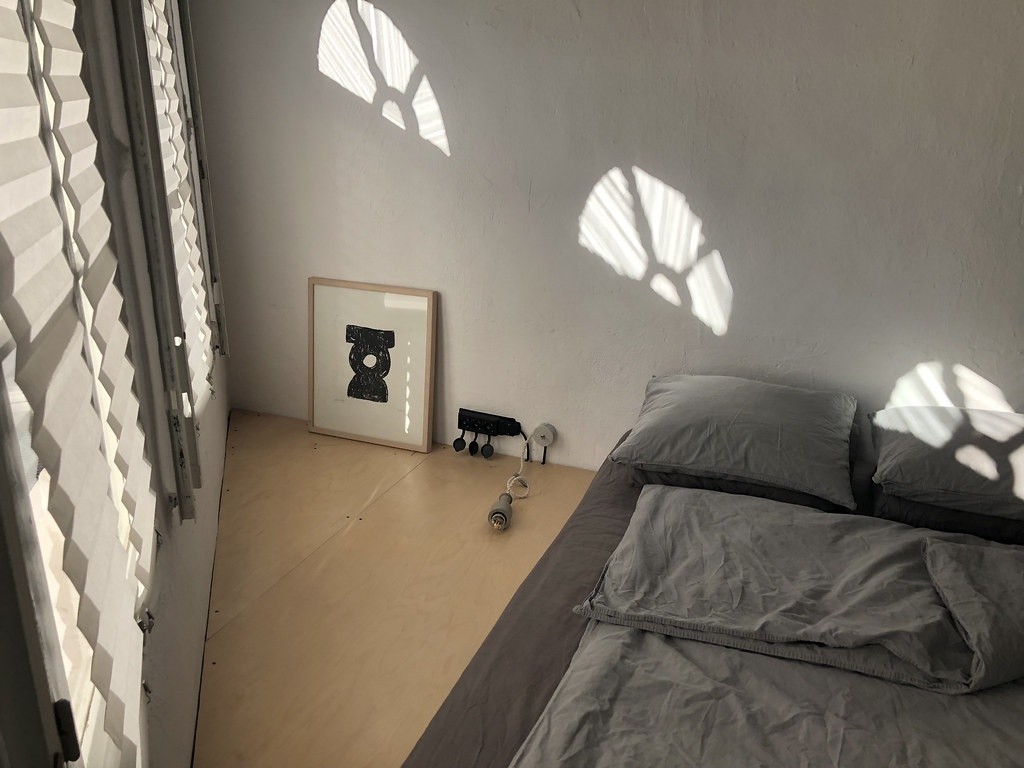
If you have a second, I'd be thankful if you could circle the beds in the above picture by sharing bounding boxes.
[400,427,1024,768]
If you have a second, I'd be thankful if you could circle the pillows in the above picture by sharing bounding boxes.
[608,374,859,511]
[867,406,1024,521]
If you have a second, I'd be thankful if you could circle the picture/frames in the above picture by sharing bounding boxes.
[305,277,437,453]
